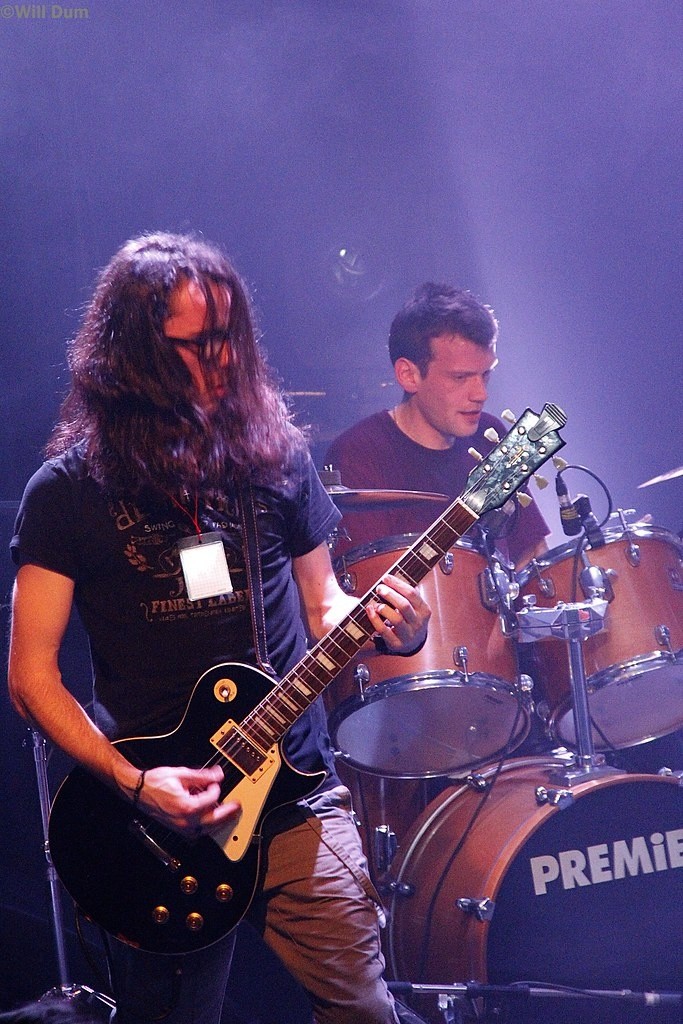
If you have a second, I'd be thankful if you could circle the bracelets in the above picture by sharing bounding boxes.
[374,621,428,657]
[131,769,147,809]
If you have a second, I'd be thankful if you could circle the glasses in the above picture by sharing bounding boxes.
[160,328,241,365]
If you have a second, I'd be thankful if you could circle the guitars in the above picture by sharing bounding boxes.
[49,399,569,958]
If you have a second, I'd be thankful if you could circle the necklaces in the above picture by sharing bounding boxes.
[393,405,397,422]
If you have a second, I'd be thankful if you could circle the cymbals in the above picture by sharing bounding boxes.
[634,465,683,491]
[323,484,446,508]
[283,390,328,398]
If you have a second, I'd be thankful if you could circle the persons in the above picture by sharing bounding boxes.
[6,233,431,1024]
[321,279,555,571]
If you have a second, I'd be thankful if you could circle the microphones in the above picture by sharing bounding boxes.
[555,474,582,536]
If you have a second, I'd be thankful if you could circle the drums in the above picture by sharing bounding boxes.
[509,522,683,753]
[381,753,682,1024]
[323,530,532,782]
